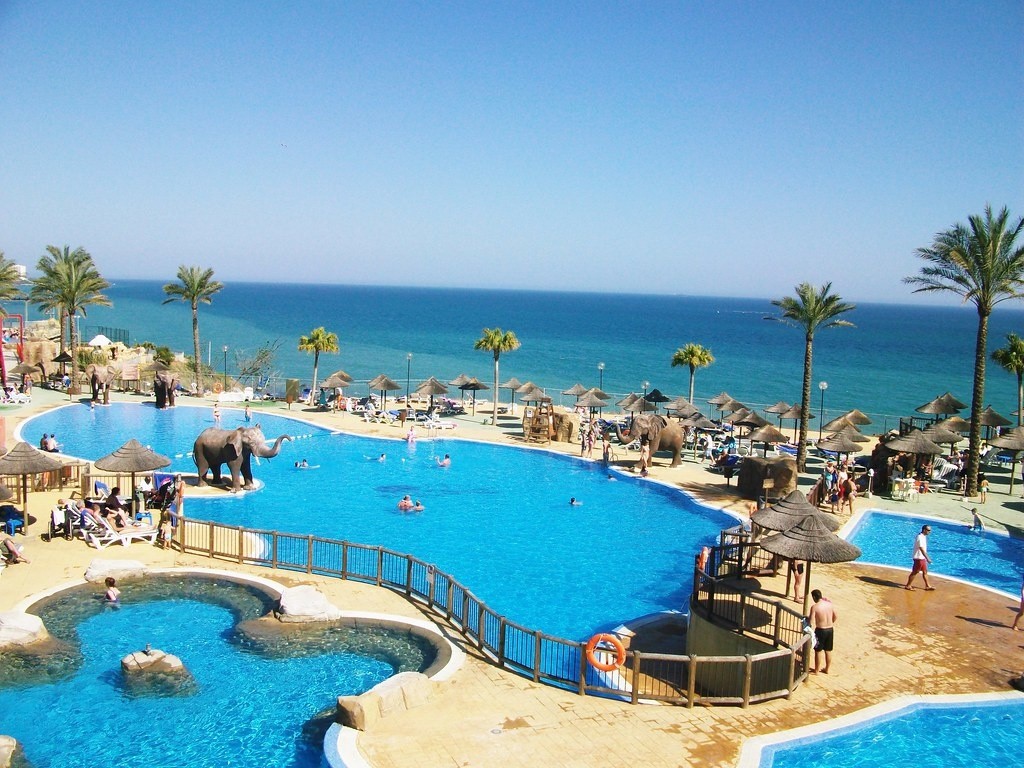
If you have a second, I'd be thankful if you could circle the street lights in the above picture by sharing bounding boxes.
[406,353,414,407]
[597,361,605,418]
[641,380,650,412]
[817,381,829,443]
[221,344,230,391]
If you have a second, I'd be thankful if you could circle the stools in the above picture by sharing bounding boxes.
[6,520,23,535]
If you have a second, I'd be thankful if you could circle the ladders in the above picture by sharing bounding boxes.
[526,397,558,446]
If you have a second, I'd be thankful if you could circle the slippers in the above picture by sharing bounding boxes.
[905,585,916,591]
[924,587,935,590]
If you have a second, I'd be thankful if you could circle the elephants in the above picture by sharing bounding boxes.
[604,413,690,476]
[191,423,292,494]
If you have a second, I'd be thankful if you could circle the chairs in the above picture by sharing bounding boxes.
[3,386,30,406]
[774,439,1012,501]
[597,418,746,475]
[70,481,159,549]
[293,390,464,425]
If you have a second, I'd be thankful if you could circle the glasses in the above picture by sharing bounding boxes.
[925,529,930,531]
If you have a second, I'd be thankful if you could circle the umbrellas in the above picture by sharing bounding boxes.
[52,351,72,375]
[142,360,171,377]
[751,489,863,617]
[93,439,171,520]
[319,363,717,461]
[708,391,1024,494]
[8,362,40,385]
[0,441,63,536]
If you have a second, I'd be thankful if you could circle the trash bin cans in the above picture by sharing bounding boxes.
[154,475,175,490]
[303,389,310,398]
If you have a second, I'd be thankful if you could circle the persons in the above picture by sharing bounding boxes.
[40,434,48,451]
[407,425,416,443]
[103,577,120,600]
[316,387,343,411]
[970,508,987,532]
[379,454,386,461]
[245,405,252,421]
[809,588,838,674]
[578,428,650,475]
[55,371,70,386]
[436,453,450,467]
[398,494,425,511]
[58,487,157,533]
[822,440,1024,515]
[13,373,34,397]
[213,403,220,422]
[0,529,31,563]
[295,459,308,467]
[905,525,938,590]
[700,431,715,464]
[47,434,62,453]
[789,559,805,603]
[1012,583,1024,631]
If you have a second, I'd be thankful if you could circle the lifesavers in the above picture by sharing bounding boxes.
[339,398,347,410]
[585,633,627,672]
[178,481,185,506]
[698,547,709,569]
[213,382,223,394]
